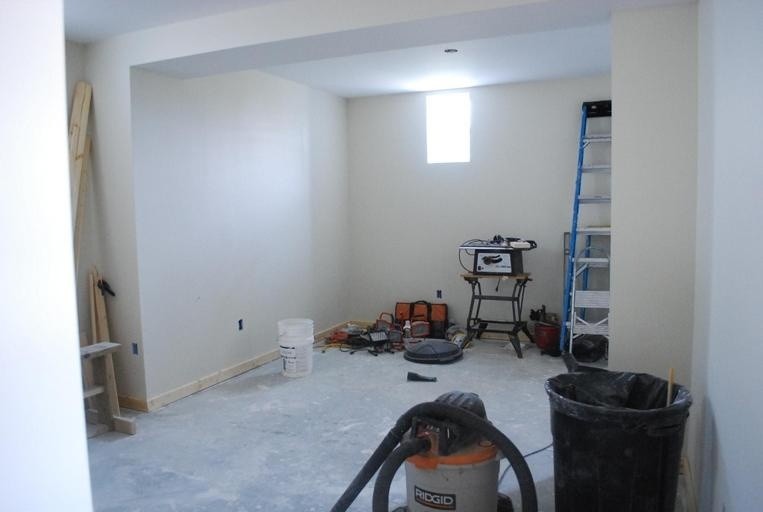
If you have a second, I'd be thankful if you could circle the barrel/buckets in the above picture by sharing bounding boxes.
[277,317,316,378]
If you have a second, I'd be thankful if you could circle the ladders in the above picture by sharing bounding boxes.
[559,100,612,353]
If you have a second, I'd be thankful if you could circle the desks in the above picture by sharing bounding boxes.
[457,271,535,361]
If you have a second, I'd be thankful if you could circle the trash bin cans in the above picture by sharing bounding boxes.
[545,371,694,512]
